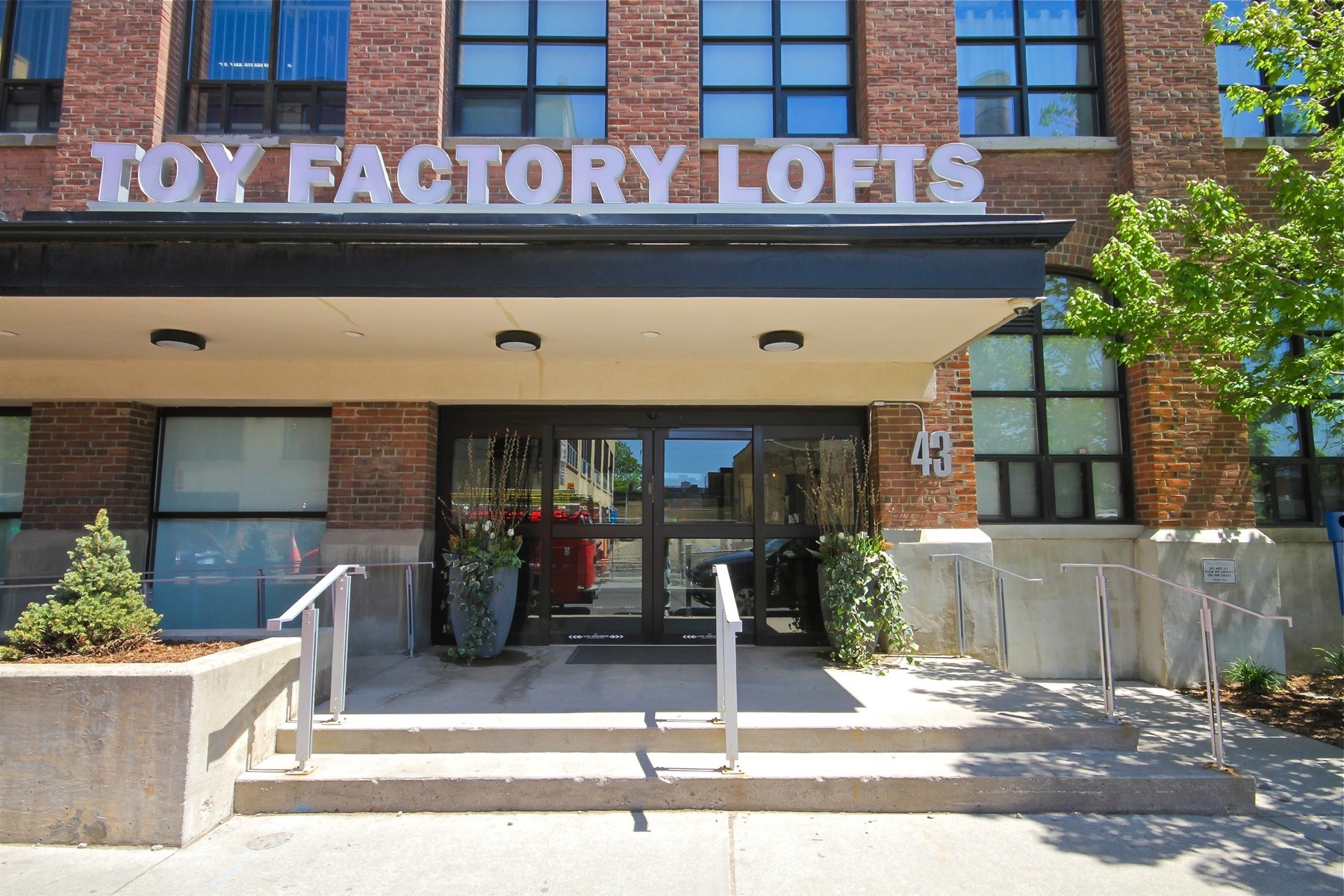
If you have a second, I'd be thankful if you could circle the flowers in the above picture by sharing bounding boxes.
[442,520,523,568]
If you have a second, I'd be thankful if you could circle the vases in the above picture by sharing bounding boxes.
[448,566,518,658]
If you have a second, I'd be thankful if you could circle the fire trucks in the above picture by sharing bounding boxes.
[446,488,602,616]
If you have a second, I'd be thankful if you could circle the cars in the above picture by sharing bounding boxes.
[691,536,799,614]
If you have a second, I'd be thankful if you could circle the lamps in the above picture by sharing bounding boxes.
[759,331,804,352]
[496,331,541,352]
[151,329,206,351]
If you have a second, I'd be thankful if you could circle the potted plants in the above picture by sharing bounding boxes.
[0,508,302,850]
[807,533,925,677]
